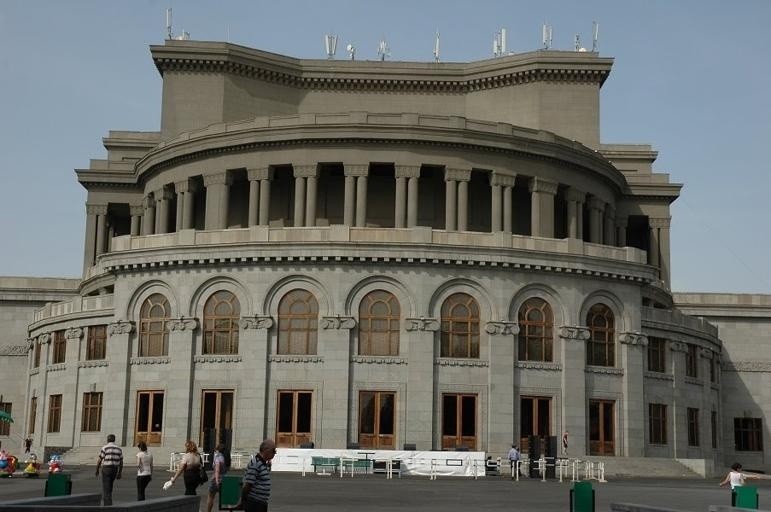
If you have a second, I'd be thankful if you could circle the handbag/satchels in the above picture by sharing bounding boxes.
[198,453,208,484]
[213,462,227,474]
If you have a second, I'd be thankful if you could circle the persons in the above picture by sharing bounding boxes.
[508,444,520,481]
[562,431,569,454]
[0,449,8,460]
[96,434,123,506]
[237,440,276,512]
[25,436,34,453]
[207,443,231,512]
[720,463,746,507]
[136,441,153,501]
[171,441,203,495]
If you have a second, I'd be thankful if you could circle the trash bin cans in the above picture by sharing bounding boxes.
[219,475,243,512]
[732,486,759,510]
[570,482,595,512]
[45,472,72,497]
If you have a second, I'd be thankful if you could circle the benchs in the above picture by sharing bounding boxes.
[311,456,402,479]
